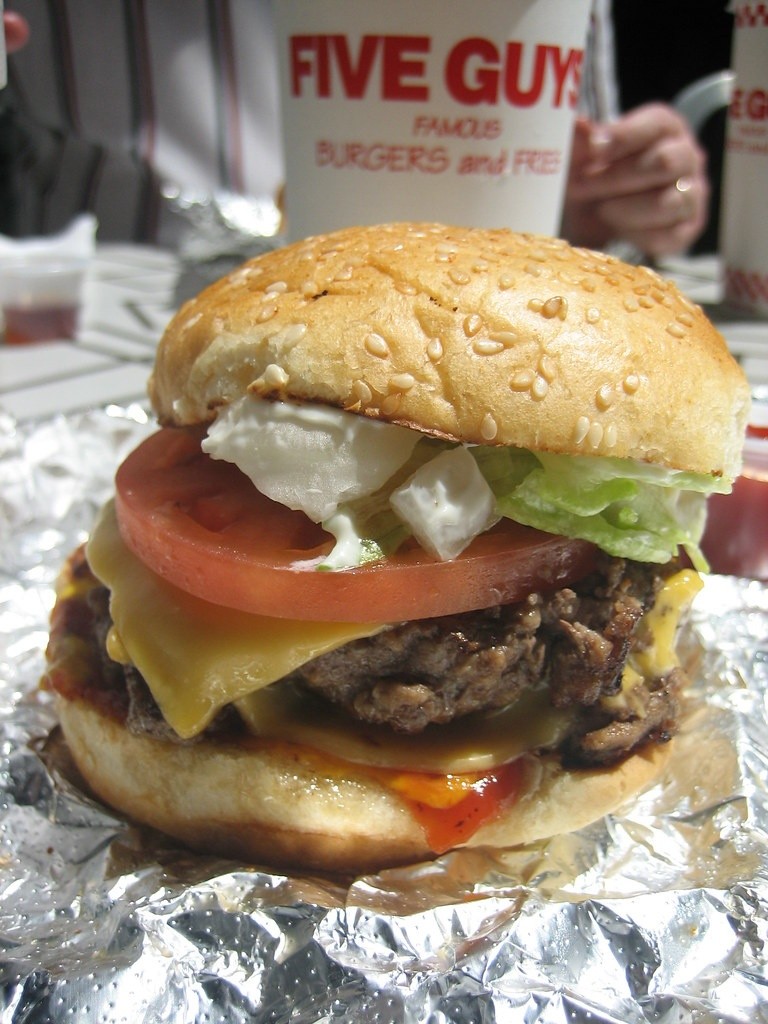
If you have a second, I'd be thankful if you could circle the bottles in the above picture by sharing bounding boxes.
[698,408,768,587]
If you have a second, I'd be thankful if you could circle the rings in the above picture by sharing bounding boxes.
[678,179,696,210]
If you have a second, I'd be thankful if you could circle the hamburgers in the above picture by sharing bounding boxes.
[42,221,751,877]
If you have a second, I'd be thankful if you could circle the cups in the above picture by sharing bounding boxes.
[717,0,767,316]
[269,0,592,246]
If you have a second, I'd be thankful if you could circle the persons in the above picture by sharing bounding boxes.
[1,1,711,261]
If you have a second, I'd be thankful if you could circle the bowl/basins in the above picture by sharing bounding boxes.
[0,257,88,350]
